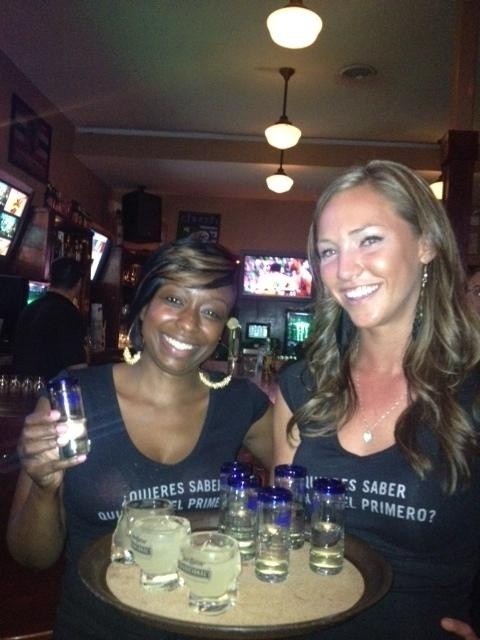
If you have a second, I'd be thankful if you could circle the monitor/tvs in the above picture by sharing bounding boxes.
[238,250,317,298]
[247,322,269,340]
[90,227,110,283]
[0,169,35,258]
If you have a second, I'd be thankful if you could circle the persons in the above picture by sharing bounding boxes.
[12,255,89,390]
[268,159,480,640]
[6,239,275,640]
[457,265,480,430]
[245,255,313,298]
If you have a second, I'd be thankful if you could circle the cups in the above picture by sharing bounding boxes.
[181,532,242,614]
[230,473,260,562]
[256,487,291,584]
[273,463,306,550]
[128,512,193,591]
[311,478,347,575]
[217,460,252,528]
[46,375,90,459]
[111,499,177,565]
[0,375,46,396]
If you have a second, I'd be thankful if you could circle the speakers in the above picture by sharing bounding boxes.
[124,191,163,242]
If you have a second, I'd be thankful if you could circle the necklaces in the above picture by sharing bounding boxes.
[355,372,409,443]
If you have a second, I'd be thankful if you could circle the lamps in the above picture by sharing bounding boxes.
[264,145,294,193]
[263,66,301,149]
[267,1,324,50]
